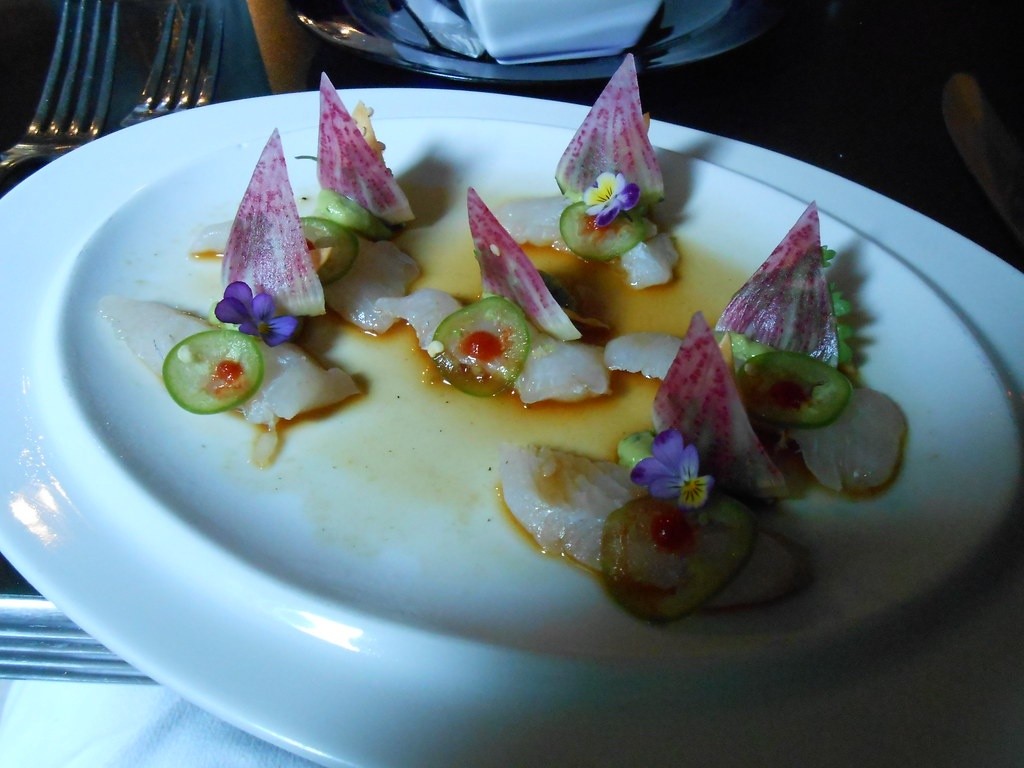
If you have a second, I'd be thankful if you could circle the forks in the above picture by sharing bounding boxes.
[122,0,222,130]
[1,1,122,173]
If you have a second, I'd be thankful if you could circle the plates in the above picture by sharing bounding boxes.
[288,1,795,96]
[1,89,1023,768]
